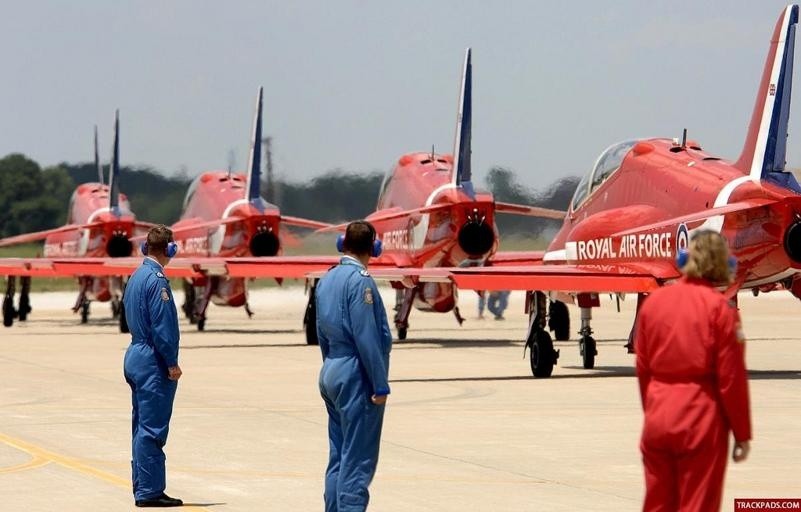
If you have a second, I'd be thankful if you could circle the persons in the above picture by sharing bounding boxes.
[123,224,186,509]
[486,289,513,321]
[313,219,393,512]
[632,229,754,511]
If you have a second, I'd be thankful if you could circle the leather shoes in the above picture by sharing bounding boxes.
[133,490,182,506]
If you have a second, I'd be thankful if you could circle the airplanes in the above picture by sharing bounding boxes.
[127,84,346,327]
[315,48,567,339]
[444,5,801,383]
[1,107,175,334]
